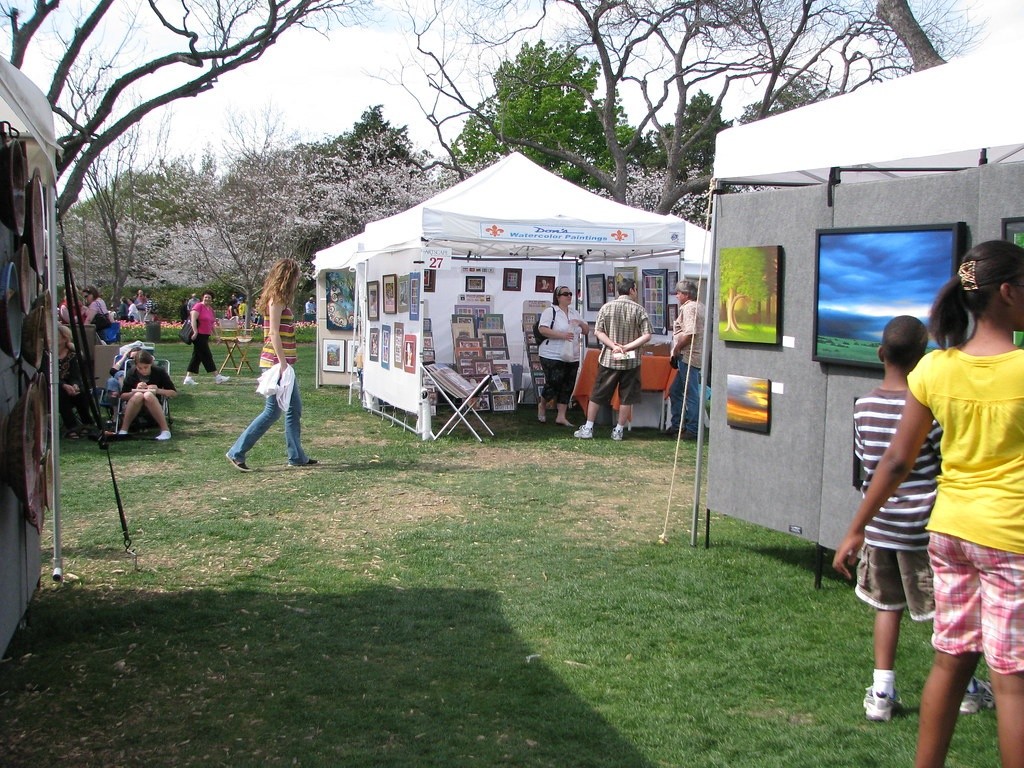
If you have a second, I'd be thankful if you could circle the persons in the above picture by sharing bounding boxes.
[226,257,318,472]
[573,277,654,441]
[57,285,316,441]
[663,281,705,440]
[831,240,1024,768]
[536,285,589,427]
[852,316,996,722]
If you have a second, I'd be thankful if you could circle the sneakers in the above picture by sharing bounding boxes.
[863,687,896,721]
[574,425,593,438]
[184,377,198,386]
[226,453,252,471]
[289,457,318,466]
[611,428,624,441]
[958,679,995,714]
[216,374,230,385]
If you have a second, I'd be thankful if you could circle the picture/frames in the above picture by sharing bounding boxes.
[502,267,522,291]
[536,276,555,292]
[423,304,518,412]
[586,266,678,335]
[323,272,420,374]
[1001,217,1024,351]
[424,270,436,291]
[466,277,486,292]
[811,221,967,369]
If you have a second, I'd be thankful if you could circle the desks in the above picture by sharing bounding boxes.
[575,349,677,431]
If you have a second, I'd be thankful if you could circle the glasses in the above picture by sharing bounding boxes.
[85,293,90,297]
[560,292,572,297]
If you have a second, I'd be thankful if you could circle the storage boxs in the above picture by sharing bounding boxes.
[643,343,671,355]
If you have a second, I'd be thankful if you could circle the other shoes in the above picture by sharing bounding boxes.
[538,403,546,422]
[156,431,171,440]
[556,420,574,427]
[119,431,127,434]
[68,433,79,440]
[677,429,697,440]
[660,426,681,437]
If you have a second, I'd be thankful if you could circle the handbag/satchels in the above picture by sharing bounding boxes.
[179,317,199,344]
[90,302,112,330]
[103,322,120,342]
[130,316,134,322]
[532,307,556,347]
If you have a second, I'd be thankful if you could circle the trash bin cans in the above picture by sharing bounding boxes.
[145,320,161,343]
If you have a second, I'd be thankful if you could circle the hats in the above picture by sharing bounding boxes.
[310,297,316,301]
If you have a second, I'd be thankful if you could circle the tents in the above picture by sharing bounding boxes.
[312,33,1024,587]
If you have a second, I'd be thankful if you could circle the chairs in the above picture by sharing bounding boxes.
[101,321,123,345]
[214,315,255,375]
[58,354,171,441]
